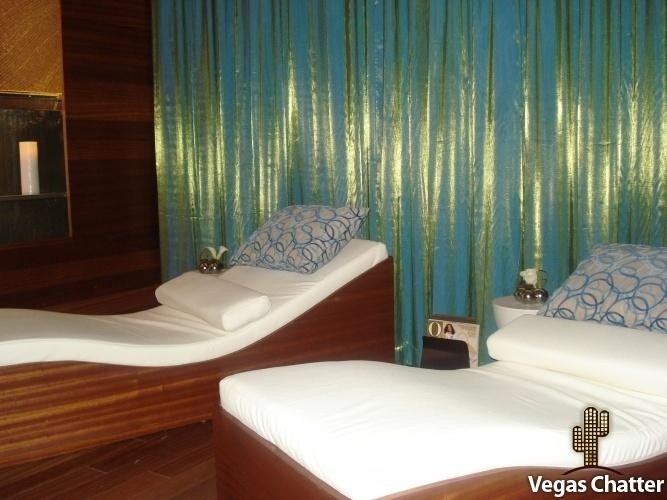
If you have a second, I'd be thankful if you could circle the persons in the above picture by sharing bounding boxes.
[442,323,456,340]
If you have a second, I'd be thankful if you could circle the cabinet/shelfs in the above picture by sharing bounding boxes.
[1,0,162,315]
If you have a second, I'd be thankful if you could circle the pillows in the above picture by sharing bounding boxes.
[154,269,271,330]
[229,205,370,275]
[485,243,667,398]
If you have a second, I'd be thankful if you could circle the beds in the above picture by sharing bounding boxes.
[215,356,667,499]
[0,238,395,500]
